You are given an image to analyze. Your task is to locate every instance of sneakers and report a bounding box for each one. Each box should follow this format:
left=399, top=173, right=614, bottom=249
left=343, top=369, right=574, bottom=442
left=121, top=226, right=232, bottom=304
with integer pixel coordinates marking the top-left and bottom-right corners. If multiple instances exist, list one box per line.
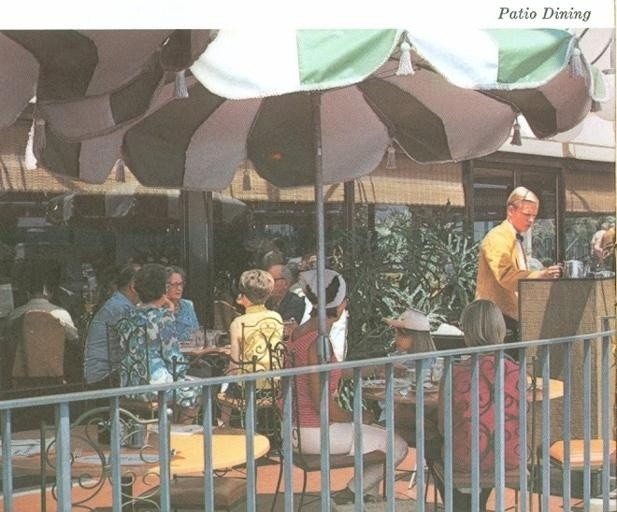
left=329, top=489, right=379, bottom=512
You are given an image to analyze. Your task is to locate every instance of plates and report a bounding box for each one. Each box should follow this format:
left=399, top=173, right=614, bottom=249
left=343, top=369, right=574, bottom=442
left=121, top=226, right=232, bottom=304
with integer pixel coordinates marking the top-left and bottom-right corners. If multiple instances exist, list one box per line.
left=359, top=370, right=439, bottom=396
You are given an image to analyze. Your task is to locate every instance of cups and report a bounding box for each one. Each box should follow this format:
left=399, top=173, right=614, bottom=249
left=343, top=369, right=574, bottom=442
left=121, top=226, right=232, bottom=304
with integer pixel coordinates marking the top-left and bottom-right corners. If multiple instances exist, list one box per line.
left=96, top=422, right=111, bottom=445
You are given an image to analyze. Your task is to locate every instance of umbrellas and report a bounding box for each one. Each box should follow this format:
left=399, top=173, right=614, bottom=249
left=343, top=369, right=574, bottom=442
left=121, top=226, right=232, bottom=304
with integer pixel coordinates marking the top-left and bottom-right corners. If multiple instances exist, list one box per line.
left=0, top=29, right=216, bottom=153
left=31, top=29, right=607, bottom=365
left=46, top=184, right=248, bottom=228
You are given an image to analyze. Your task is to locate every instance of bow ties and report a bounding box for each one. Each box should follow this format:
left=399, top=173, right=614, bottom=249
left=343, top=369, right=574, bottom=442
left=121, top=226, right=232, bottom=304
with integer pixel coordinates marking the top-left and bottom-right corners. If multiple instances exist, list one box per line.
left=515, top=232, right=525, bottom=242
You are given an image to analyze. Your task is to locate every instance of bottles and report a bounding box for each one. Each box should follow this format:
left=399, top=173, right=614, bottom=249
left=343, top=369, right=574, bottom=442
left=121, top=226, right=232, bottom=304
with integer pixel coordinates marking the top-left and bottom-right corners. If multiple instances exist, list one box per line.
left=431, top=358, right=445, bottom=384
left=128, top=414, right=147, bottom=449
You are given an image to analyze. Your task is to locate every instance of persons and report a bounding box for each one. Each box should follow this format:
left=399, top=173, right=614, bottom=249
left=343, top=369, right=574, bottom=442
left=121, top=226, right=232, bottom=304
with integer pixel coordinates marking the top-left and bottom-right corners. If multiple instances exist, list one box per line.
left=473, top=186, right=561, bottom=362
left=380, top=307, right=439, bottom=447
left=424, top=299, right=530, bottom=511
left=589, top=221, right=615, bottom=272
left=1, top=259, right=306, bottom=425
left=286, top=268, right=407, bottom=504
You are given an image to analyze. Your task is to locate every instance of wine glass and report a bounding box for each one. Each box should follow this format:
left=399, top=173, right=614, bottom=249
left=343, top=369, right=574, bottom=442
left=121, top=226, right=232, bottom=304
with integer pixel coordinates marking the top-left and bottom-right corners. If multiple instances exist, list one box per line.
left=183, top=325, right=217, bottom=352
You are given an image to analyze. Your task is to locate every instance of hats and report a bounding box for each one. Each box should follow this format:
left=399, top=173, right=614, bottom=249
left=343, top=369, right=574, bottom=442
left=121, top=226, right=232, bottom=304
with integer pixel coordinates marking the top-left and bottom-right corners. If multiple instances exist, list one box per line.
left=298, top=265, right=349, bottom=312
left=379, top=308, right=433, bottom=334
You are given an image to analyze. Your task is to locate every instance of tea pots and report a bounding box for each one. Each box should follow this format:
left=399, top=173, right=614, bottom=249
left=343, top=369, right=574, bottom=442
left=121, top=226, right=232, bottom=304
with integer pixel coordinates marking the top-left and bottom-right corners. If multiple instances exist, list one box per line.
left=553, top=260, right=590, bottom=278
